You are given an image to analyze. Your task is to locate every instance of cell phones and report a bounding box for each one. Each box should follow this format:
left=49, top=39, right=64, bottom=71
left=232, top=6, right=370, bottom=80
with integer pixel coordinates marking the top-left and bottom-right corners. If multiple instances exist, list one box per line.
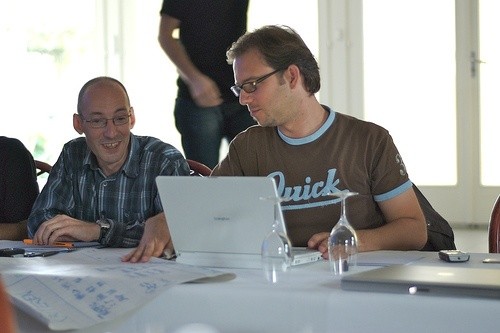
left=439, top=250, right=470, bottom=262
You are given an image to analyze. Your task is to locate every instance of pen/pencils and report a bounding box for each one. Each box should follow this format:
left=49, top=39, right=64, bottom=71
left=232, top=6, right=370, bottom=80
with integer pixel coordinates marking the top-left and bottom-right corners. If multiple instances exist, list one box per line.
left=22, top=239, right=74, bottom=247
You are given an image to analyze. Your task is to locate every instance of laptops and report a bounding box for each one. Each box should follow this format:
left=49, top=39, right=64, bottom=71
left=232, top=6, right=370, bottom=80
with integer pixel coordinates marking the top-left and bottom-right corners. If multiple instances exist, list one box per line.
left=340, top=264, right=500, bottom=299
left=156, top=175, right=325, bottom=267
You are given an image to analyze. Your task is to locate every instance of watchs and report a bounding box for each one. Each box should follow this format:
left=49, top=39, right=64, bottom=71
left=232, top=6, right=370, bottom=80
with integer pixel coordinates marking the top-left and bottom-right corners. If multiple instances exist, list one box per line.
left=96, top=218, right=110, bottom=243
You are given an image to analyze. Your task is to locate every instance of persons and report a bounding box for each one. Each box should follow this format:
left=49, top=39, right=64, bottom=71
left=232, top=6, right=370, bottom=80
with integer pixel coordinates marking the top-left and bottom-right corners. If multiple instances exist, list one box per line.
left=120, top=25, right=428, bottom=263
left=0, top=135, right=39, bottom=240
left=26, top=76, right=189, bottom=246
left=158, top=0, right=261, bottom=175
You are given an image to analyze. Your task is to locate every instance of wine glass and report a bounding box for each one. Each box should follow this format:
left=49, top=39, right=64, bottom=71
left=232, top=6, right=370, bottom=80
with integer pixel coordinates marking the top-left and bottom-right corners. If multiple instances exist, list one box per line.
left=257, top=195, right=294, bottom=270
left=322, top=191, right=360, bottom=277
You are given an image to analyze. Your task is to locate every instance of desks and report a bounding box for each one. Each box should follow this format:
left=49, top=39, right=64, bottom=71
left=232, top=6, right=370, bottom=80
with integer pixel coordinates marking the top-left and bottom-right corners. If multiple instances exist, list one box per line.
left=0, top=240, right=500, bottom=333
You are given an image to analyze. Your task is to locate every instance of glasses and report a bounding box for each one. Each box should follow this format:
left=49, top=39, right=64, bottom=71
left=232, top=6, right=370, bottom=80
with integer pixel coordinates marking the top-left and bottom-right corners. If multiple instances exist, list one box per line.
left=230, top=67, right=290, bottom=97
left=81, top=110, right=132, bottom=128
